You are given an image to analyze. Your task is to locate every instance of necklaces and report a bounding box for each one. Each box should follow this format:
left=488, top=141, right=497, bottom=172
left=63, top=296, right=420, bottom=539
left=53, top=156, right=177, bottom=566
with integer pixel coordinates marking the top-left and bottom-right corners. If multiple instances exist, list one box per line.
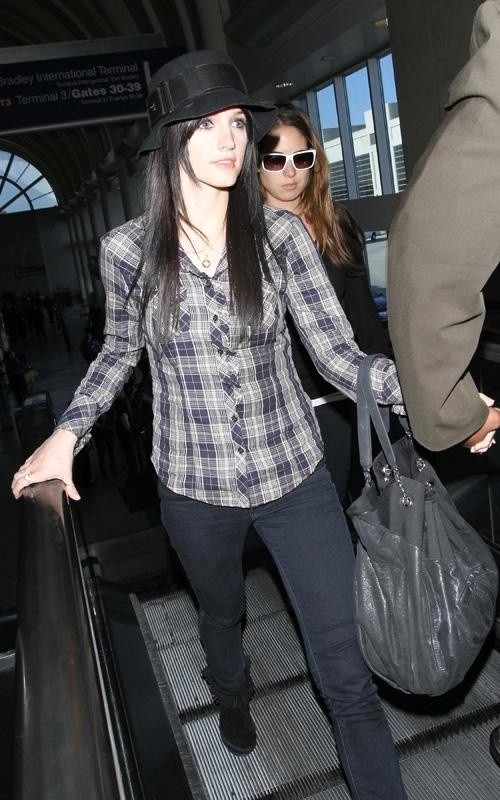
left=200, top=228, right=223, bottom=269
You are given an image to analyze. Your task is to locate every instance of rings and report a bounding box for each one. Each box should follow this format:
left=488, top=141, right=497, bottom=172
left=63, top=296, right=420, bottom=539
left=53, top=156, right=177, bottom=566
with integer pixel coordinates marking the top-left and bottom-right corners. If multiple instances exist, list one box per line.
left=24, top=472, right=33, bottom=482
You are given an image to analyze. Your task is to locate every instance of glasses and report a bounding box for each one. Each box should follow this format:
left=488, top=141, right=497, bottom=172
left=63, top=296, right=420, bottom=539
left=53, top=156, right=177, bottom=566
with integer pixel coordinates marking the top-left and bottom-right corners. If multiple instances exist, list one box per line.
left=259, top=147, right=319, bottom=174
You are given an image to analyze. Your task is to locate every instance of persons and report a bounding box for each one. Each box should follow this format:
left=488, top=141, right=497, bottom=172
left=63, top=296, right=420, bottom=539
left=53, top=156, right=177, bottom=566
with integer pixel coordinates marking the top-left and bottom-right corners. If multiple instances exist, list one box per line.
left=382, top=1, right=499, bottom=767
left=80, top=362, right=153, bottom=488
left=257, top=100, right=390, bottom=510
left=80, top=331, right=103, bottom=365
left=11, top=47, right=497, bottom=799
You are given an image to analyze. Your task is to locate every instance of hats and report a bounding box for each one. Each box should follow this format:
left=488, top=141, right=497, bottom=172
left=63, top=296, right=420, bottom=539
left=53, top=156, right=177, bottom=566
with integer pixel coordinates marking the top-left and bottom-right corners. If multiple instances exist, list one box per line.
left=133, top=46, right=278, bottom=161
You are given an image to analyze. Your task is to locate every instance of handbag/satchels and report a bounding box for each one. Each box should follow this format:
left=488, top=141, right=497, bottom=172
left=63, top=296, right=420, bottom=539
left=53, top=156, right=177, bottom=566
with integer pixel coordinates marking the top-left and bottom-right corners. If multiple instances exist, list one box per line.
left=346, top=351, right=498, bottom=700
